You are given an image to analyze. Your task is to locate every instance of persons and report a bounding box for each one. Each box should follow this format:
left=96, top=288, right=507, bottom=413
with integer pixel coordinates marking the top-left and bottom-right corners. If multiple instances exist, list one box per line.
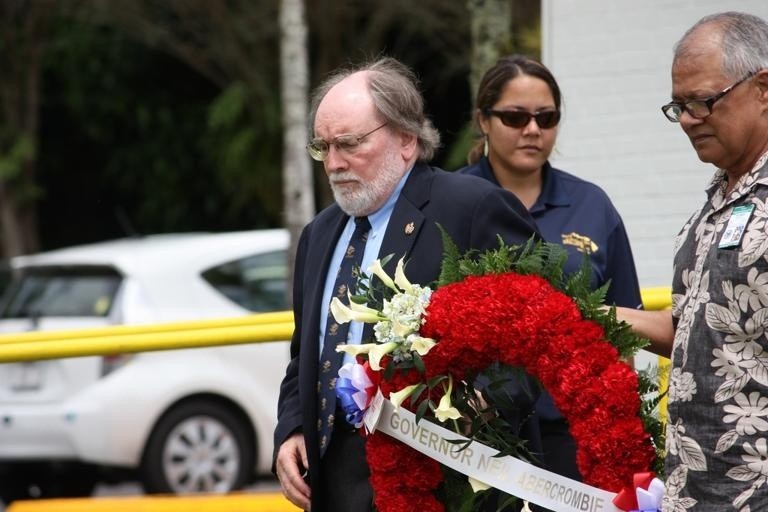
left=448, top=55, right=645, bottom=512
left=575, top=14, right=768, bottom=512
left=273, top=60, right=584, bottom=512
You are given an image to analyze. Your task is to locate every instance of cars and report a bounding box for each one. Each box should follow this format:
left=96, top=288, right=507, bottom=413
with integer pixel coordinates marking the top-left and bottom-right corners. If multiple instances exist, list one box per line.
left=0, top=229, right=290, bottom=498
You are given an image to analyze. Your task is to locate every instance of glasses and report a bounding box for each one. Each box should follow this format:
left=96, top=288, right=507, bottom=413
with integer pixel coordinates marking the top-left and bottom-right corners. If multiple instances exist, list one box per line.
left=661, top=72, right=757, bottom=123
left=305, top=121, right=390, bottom=161
left=488, top=109, right=562, bottom=129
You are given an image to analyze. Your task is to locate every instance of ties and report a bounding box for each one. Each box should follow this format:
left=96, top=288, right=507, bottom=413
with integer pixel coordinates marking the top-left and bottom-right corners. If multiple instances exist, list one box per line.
left=318, top=215, right=372, bottom=458
